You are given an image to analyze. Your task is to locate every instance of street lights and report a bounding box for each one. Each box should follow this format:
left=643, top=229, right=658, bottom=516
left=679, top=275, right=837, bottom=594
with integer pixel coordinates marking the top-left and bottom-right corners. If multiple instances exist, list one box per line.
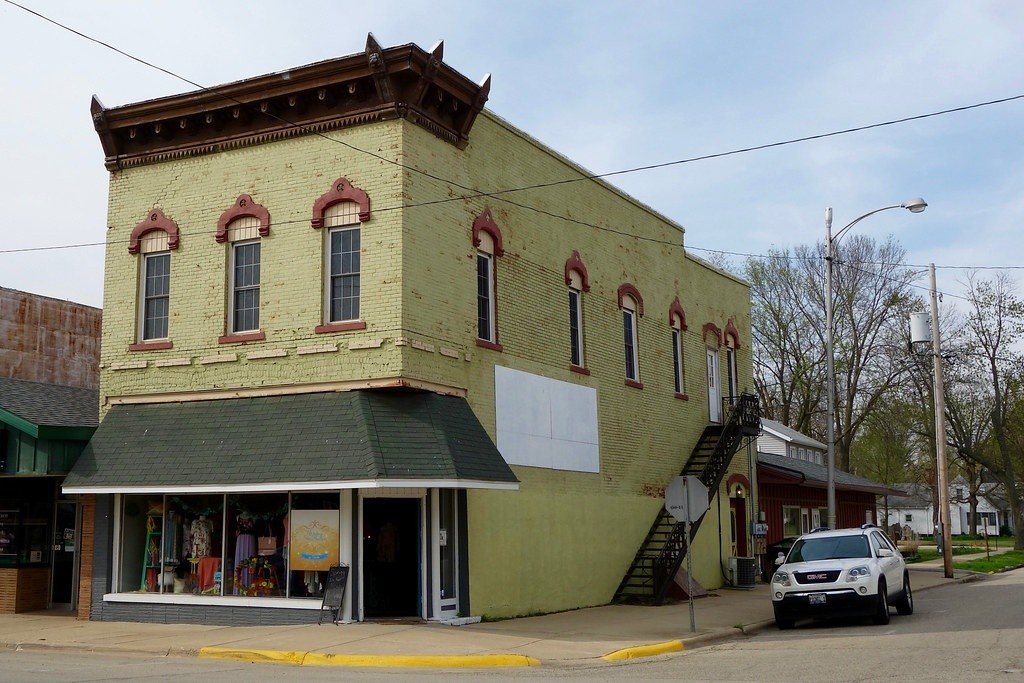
left=826, top=198, right=928, bottom=532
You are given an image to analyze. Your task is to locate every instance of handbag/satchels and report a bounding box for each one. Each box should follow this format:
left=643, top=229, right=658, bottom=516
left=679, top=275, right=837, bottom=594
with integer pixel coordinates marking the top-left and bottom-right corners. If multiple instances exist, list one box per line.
left=258, top=522, right=277, bottom=556
left=158, top=572, right=177, bottom=585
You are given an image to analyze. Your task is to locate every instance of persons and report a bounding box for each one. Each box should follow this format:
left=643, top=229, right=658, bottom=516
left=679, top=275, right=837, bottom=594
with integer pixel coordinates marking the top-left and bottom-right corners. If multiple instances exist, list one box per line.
left=233, top=510, right=256, bottom=595
left=182, top=518, right=192, bottom=558
left=191, top=515, right=213, bottom=558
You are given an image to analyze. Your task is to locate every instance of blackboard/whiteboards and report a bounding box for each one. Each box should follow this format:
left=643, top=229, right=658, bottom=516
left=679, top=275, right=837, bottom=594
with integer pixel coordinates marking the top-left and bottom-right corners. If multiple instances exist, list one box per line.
left=322, top=567, right=350, bottom=606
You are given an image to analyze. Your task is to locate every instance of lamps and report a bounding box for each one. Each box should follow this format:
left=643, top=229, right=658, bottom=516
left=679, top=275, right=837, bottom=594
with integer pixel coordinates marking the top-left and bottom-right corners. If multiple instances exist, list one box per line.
left=737, top=488, right=742, bottom=494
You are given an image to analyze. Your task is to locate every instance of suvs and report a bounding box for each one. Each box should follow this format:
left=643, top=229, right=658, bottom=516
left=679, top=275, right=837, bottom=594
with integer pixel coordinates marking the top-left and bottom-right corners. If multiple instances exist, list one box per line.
left=770, top=522, right=913, bottom=631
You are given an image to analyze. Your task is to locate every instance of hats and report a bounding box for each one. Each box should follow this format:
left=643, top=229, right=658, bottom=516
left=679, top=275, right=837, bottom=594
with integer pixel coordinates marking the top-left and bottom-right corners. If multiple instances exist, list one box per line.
left=240, top=512, right=252, bottom=519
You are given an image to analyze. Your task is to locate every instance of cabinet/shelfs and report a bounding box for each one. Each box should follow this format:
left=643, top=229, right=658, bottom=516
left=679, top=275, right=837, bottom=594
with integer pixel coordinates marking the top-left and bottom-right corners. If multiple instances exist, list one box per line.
left=0, top=508, right=24, bottom=563
